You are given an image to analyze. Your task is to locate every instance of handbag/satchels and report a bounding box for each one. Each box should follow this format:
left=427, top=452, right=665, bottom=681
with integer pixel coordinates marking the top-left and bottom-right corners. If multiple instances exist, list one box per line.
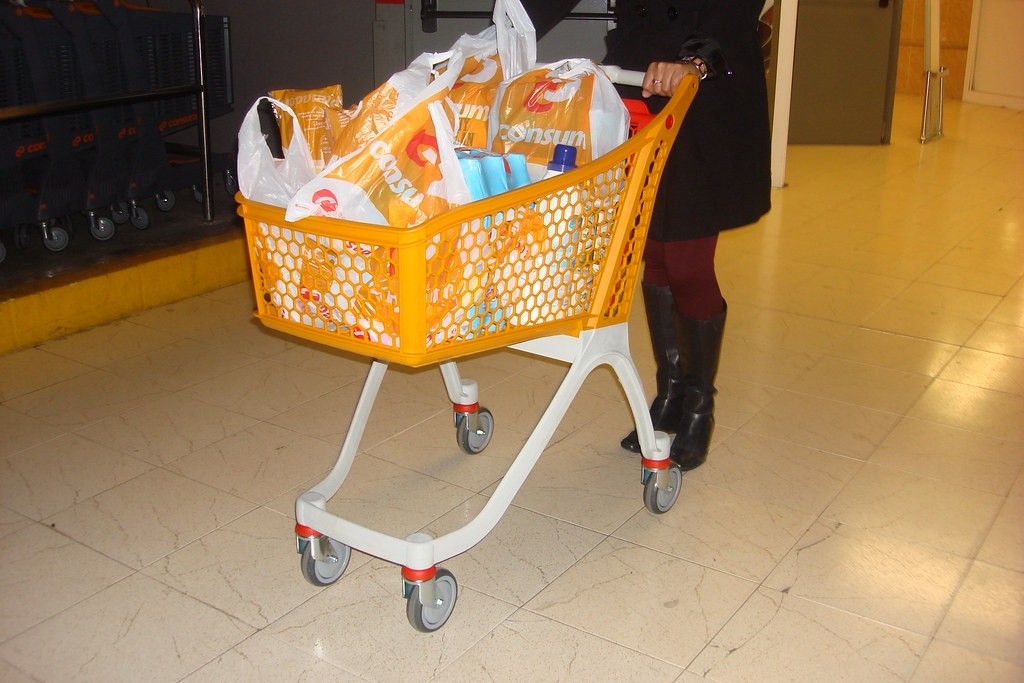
left=238, top=0, right=631, bottom=350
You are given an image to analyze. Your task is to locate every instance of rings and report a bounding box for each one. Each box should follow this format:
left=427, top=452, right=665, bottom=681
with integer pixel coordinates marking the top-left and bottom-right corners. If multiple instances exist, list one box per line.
left=652, top=79, right=662, bottom=85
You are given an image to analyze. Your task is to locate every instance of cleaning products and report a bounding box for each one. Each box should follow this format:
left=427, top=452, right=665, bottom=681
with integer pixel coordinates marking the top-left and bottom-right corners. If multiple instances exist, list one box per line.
left=526, top=143, right=589, bottom=327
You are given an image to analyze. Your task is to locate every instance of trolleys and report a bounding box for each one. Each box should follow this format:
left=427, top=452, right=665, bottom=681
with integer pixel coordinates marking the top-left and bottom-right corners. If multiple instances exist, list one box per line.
left=234, top=62, right=700, bottom=635
left=1, top=0, right=242, bottom=262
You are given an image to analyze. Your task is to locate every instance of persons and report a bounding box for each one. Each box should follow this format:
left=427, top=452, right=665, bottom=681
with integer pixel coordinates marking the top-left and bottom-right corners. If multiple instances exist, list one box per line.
left=520, top=0, right=773, bottom=469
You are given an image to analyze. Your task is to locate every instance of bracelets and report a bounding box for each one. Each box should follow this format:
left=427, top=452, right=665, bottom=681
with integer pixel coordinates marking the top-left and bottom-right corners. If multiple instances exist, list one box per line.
left=681, top=55, right=708, bottom=80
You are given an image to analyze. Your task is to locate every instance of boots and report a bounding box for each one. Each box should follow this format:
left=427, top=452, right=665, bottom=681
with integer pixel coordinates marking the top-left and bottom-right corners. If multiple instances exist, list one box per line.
left=670, top=298, right=728, bottom=472
left=620, top=282, right=682, bottom=452
